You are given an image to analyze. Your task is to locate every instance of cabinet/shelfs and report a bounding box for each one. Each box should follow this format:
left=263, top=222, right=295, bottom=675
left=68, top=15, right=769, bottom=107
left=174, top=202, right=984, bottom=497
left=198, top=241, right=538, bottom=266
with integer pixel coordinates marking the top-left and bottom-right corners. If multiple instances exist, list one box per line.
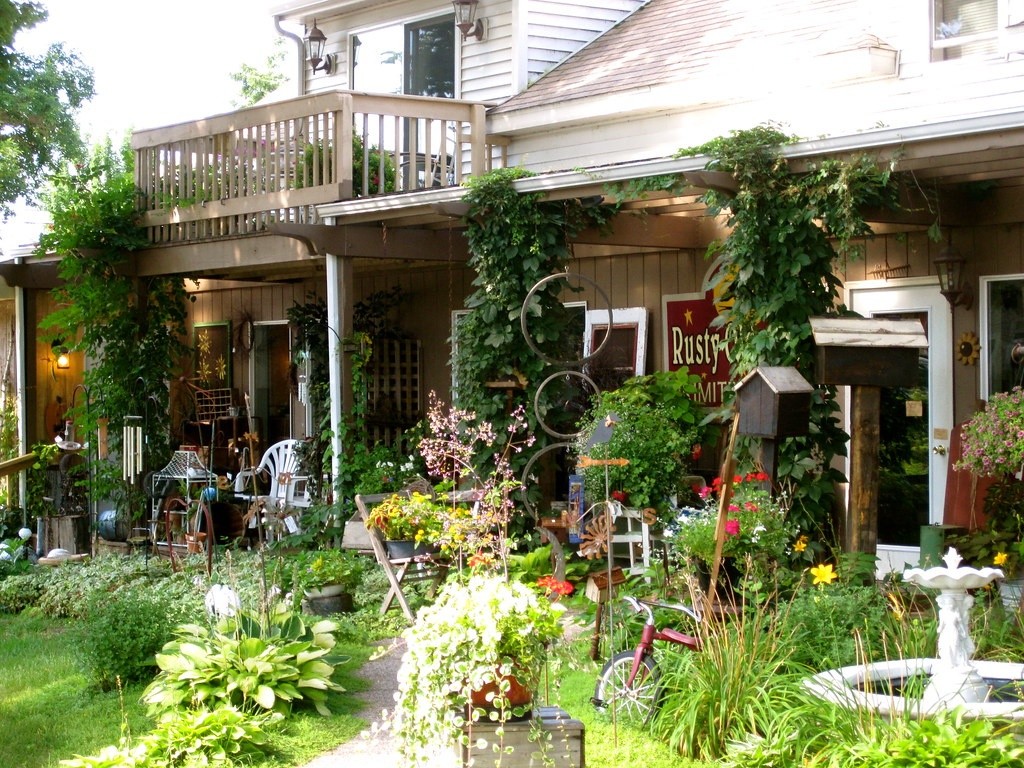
left=605, top=504, right=664, bottom=588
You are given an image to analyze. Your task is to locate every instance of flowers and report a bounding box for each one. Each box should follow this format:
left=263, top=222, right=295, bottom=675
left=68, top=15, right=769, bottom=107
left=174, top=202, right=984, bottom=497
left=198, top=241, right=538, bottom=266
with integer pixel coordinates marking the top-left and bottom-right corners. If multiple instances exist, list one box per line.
left=946, top=384, right=1024, bottom=478
left=366, top=490, right=467, bottom=554
left=678, top=470, right=792, bottom=558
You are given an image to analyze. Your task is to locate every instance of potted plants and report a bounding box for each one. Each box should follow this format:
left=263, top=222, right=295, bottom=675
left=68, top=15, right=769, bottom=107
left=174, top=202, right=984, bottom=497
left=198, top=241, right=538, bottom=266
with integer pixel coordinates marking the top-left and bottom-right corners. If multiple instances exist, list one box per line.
left=75, top=456, right=137, bottom=542
left=540, top=508, right=568, bottom=543
left=229, top=400, right=240, bottom=416
left=980, top=475, right=1024, bottom=567
left=361, top=570, right=572, bottom=768
left=297, top=553, right=367, bottom=615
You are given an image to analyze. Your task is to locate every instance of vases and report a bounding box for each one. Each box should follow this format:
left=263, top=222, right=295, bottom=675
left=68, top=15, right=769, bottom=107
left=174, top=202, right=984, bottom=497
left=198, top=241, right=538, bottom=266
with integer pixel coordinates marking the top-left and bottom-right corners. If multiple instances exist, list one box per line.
left=187, top=532, right=207, bottom=553
left=383, top=539, right=440, bottom=559
left=696, top=554, right=746, bottom=603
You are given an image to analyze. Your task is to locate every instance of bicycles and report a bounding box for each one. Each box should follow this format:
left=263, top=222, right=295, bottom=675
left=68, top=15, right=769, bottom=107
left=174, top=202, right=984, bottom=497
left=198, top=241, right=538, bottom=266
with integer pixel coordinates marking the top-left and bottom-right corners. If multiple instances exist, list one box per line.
left=589, top=595, right=786, bottom=744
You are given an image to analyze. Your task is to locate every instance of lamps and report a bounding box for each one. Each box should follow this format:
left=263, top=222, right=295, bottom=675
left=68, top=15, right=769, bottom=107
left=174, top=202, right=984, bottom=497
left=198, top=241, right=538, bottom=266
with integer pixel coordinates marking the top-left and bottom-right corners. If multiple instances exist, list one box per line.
left=57, top=383, right=111, bottom=563
left=933, top=242, right=974, bottom=313
left=49, top=333, right=70, bottom=382
left=451, top=0, right=489, bottom=44
left=304, top=17, right=335, bottom=77
left=806, top=33, right=902, bottom=87
left=117, top=375, right=149, bottom=557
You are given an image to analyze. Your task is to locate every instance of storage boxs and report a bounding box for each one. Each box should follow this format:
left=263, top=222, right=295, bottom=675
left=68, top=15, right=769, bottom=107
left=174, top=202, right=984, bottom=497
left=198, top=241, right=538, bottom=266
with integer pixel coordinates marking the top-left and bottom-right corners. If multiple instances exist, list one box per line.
left=450, top=704, right=585, bottom=768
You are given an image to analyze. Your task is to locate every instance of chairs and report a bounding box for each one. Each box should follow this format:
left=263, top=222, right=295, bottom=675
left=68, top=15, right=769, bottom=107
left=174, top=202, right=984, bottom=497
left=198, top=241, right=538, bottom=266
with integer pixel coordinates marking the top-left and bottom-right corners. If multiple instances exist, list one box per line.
left=285, top=453, right=414, bottom=539
left=388, top=116, right=458, bottom=191
left=233, top=438, right=303, bottom=544
